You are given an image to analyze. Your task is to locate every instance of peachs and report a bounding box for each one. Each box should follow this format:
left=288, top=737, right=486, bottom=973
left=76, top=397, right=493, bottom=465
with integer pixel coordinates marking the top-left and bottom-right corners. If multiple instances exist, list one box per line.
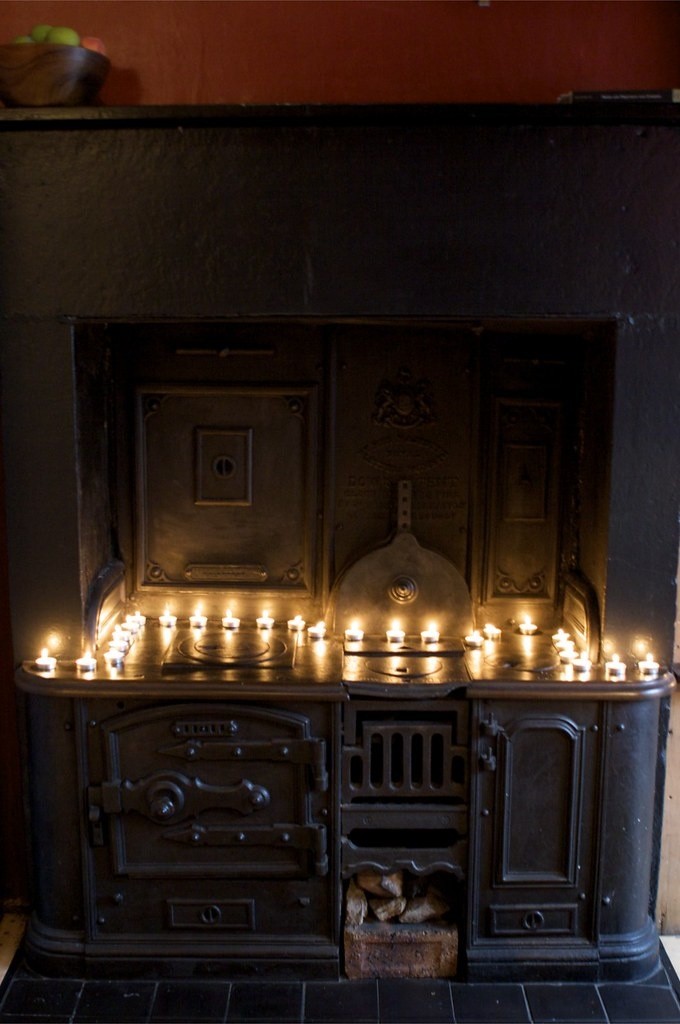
left=78, top=37, right=107, bottom=56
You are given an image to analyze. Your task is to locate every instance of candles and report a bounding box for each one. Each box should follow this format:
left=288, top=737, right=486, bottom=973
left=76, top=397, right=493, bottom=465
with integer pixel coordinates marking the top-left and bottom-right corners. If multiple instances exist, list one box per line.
left=638, top=653, right=659, bottom=674
left=345, top=621, right=363, bottom=641
left=104, top=612, right=146, bottom=665
left=420, top=623, right=439, bottom=643
left=189, top=610, right=207, bottom=627
left=35, top=648, right=56, bottom=670
left=605, top=653, right=626, bottom=677
left=386, top=620, right=405, bottom=643
left=76, top=650, right=96, bottom=670
left=519, top=617, right=536, bottom=635
left=288, top=615, right=305, bottom=630
left=222, top=610, right=240, bottom=628
left=159, top=611, right=177, bottom=626
left=484, top=623, right=501, bottom=639
left=255, top=611, right=274, bottom=629
left=308, top=621, right=326, bottom=637
left=552, top=628, right=591, bottom=672
left=465, top=630, right=484, bottom=646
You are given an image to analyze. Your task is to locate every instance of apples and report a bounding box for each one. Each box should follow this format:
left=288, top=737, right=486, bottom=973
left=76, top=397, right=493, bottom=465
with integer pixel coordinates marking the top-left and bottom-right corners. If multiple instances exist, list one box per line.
left=12, top=22, right=81, bottom=46
